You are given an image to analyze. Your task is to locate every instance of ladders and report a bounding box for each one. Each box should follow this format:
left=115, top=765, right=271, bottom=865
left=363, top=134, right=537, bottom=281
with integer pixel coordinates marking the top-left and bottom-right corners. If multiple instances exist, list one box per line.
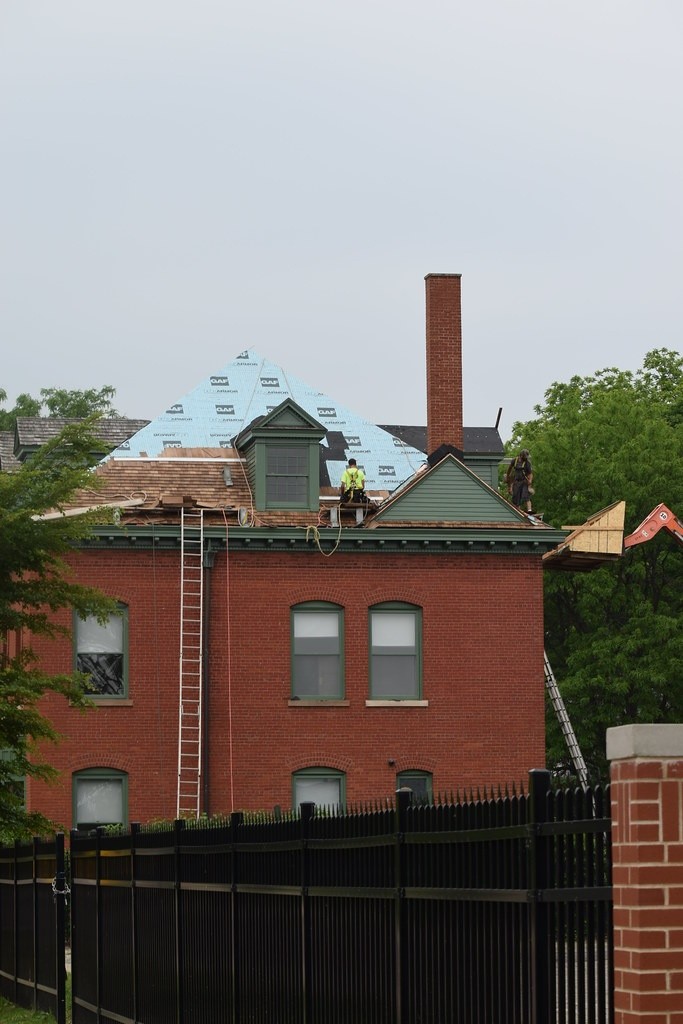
left=544, top=649, right=597, bottom=818
left=177, top=506, right=203, bottom=820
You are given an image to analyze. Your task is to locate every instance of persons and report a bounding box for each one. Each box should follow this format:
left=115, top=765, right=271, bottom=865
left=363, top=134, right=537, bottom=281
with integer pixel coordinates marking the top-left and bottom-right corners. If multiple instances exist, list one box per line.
left=340, top=459, right=365, bottom=501
left=507, top=449, right=533, bottom=514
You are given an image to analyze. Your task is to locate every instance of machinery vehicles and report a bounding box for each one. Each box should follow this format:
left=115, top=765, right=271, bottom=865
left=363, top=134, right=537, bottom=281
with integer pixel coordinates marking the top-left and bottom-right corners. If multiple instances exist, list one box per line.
left=543, top=503, right=683, bottom=569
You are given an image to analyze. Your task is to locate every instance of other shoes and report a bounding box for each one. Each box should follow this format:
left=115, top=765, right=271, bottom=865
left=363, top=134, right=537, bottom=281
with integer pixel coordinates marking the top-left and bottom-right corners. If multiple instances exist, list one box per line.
left=528, top=510, right=537, bottom=515
